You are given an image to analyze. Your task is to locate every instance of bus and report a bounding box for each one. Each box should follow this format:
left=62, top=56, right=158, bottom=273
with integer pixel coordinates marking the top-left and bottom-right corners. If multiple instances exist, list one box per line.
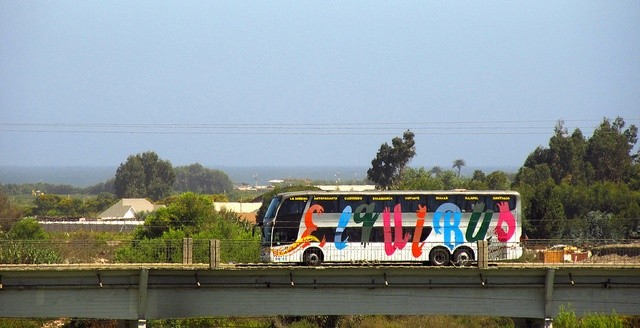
left=251, top=189, right=524, bottom=266
